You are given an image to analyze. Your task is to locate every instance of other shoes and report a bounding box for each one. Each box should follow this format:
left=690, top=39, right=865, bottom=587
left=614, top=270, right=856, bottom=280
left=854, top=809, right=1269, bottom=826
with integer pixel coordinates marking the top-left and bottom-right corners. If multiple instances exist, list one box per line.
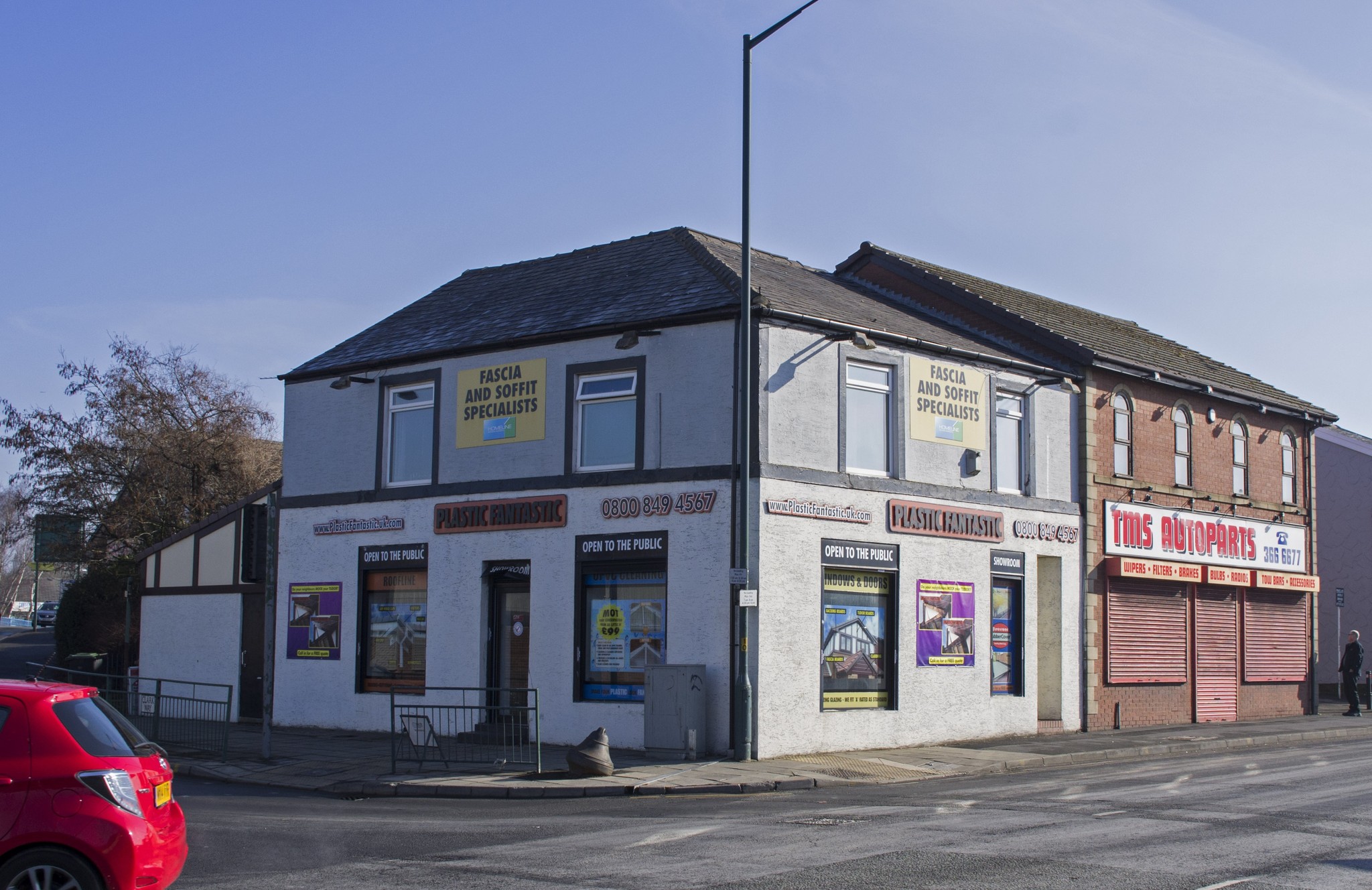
left=1342, top=709, right=1362, bottom=717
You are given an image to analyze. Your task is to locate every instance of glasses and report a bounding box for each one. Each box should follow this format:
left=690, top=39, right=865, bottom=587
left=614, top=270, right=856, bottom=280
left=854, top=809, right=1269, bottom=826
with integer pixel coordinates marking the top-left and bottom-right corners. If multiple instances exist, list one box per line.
left=1348, top=633, right=1354, bottom=636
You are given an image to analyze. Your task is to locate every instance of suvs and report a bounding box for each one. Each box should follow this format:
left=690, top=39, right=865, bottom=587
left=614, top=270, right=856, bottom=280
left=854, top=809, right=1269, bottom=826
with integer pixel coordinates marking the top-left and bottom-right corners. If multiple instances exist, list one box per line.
left=31, top=600, right=60, bottom=627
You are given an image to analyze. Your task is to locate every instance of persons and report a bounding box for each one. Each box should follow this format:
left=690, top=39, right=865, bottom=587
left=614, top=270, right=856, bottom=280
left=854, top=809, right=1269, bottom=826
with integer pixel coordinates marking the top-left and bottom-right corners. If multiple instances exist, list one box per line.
left=1337, top=630, right=1364, bottom=716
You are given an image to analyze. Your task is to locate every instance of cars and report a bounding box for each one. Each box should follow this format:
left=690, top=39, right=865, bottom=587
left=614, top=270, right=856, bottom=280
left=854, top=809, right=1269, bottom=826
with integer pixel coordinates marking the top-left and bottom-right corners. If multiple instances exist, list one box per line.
left=0, top=676, right=186, bottom=890
